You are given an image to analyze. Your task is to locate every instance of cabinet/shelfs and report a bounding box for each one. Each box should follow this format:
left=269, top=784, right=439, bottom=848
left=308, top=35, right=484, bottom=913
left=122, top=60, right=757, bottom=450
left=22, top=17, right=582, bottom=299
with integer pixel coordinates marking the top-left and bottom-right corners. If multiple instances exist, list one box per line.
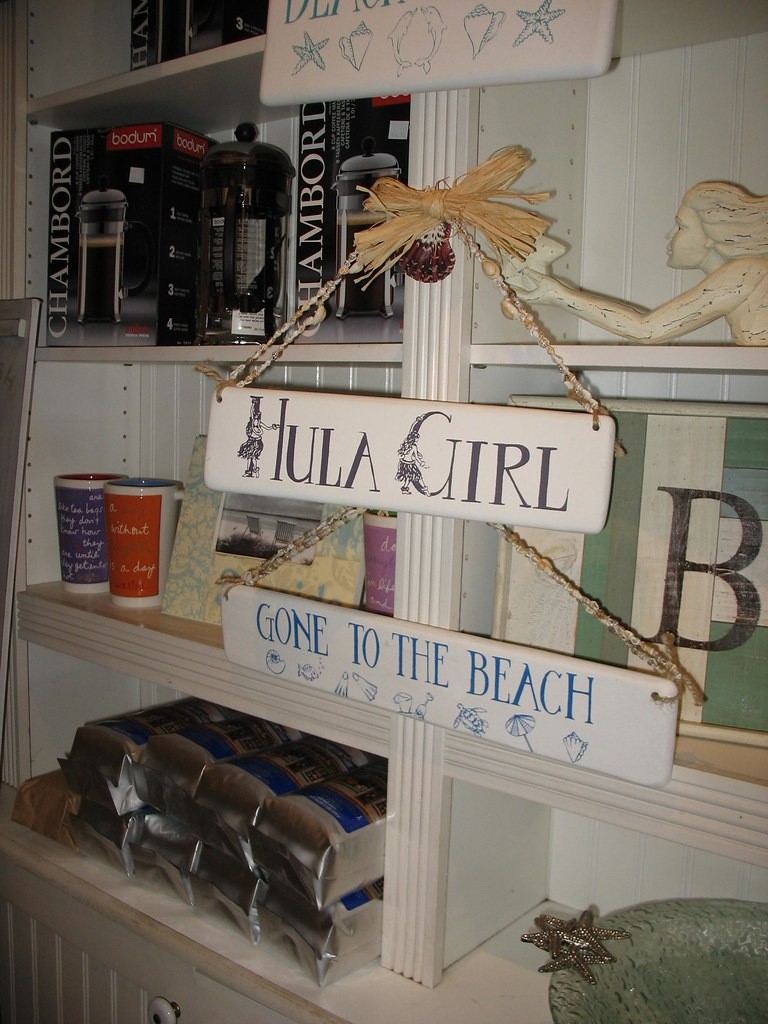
left=0, top=0, right=768, bottom=1024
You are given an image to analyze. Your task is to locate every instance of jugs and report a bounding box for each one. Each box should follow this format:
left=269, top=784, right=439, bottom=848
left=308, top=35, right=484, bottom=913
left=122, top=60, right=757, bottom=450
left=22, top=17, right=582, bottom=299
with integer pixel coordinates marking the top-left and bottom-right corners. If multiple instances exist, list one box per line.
left=193, top=122, right=296, bottom=344
left=332, top=150, right=402, bottom=319
left=75, top=187, right=127, bottom=324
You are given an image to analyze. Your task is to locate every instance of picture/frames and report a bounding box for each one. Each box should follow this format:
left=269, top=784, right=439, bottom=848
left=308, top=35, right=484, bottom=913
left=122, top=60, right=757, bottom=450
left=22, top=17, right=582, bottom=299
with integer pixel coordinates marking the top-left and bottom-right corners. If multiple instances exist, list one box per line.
left=162, top=436, right=367, bottom=626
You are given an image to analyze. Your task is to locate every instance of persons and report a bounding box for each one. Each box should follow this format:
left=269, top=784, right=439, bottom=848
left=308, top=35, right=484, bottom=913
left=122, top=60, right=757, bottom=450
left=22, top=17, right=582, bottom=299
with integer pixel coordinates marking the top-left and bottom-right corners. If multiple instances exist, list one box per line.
left=511, top=179, right=768, bottom=344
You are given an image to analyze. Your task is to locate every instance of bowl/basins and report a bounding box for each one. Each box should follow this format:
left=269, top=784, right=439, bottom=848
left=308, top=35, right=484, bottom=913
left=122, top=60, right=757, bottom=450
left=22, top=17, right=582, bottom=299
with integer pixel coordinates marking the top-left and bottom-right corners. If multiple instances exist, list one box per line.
left=549, top=896, right=768, bottom=1024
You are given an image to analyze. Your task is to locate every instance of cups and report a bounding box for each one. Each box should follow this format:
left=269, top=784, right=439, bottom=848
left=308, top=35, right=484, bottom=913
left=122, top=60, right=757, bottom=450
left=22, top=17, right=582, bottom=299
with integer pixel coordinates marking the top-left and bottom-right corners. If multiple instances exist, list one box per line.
left=363, top=510, right=398, bottom=617
left=103, top=478, right=186, bottom=610
left=54, top=472, right=130, bottom=592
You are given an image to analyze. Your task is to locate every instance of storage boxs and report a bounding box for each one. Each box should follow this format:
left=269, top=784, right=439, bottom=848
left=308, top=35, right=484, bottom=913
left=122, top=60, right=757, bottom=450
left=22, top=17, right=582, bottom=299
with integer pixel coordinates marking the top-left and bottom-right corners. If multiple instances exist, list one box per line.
left=186, top=0, right=269, bottom=54
left=295, top=93, right=410, bottom=344
left=45, top=121, right=220, bottom=345
left=131, top=0, right=187, bottom=72
